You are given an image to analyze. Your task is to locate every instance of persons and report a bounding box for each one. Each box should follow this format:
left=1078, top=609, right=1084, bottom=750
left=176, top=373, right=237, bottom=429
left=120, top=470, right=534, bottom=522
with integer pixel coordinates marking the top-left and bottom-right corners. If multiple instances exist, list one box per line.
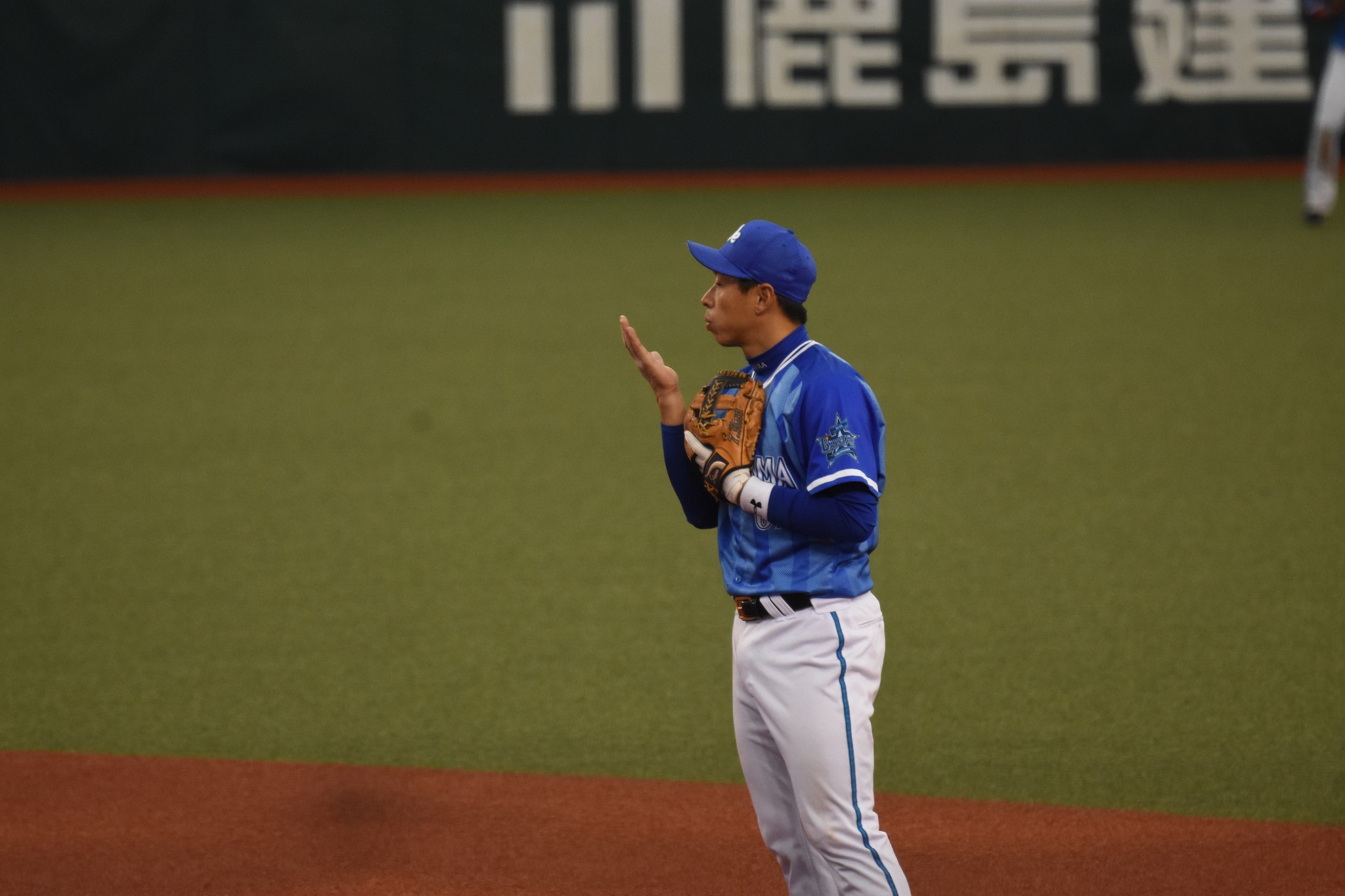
left=1304, top=1, right=1345, bottom=224
left=620, top=220, right=915, bottom=896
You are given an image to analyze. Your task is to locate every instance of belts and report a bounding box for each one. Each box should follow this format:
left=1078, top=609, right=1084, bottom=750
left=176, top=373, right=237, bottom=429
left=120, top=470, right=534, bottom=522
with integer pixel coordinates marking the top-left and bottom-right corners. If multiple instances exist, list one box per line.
left=735, top=594, right=813, bottom=621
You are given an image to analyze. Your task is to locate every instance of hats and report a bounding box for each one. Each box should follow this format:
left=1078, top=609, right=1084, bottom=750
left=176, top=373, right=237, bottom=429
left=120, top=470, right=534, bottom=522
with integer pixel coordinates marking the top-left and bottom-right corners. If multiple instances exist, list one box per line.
left=687, top=220, right=817, bottom=303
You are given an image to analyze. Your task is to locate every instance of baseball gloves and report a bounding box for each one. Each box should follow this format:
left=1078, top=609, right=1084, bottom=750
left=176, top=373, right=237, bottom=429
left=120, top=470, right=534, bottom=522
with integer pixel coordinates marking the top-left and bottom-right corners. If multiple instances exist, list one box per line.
left=683, top=368, right=767, bottom=503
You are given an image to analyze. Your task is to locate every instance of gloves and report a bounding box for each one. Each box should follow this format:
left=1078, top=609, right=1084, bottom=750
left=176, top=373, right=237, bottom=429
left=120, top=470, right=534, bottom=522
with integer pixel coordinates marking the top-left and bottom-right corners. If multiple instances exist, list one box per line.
left=684, top=430, right=750, bottom=504
